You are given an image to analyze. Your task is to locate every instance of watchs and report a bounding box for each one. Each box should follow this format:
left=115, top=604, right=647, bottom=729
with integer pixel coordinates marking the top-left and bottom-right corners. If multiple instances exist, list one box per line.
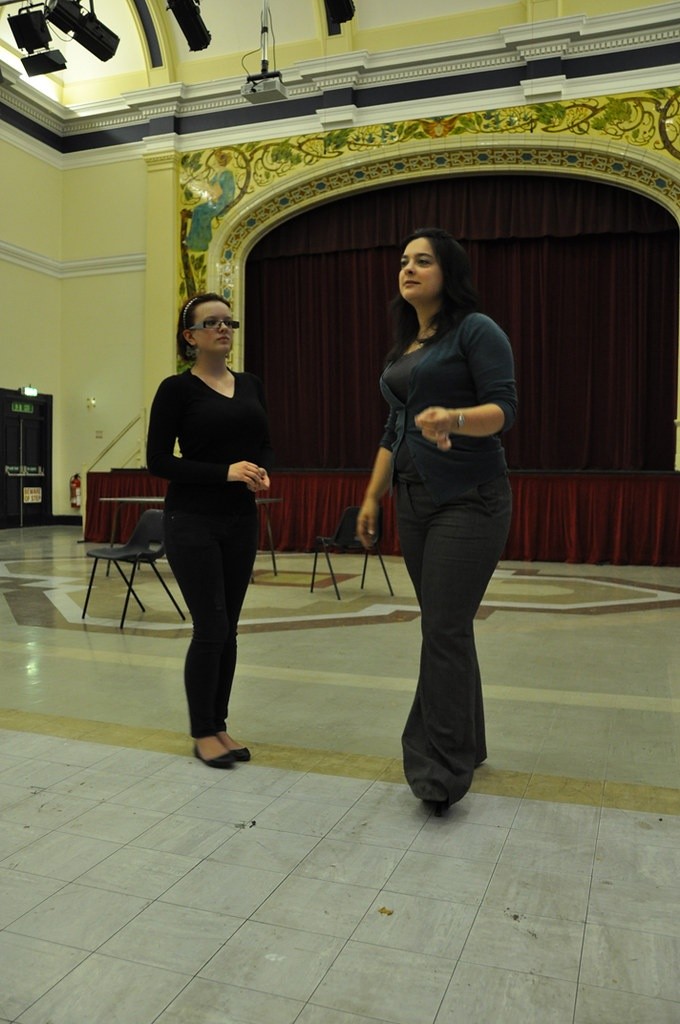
left=452, top=408, right=465, bottom=431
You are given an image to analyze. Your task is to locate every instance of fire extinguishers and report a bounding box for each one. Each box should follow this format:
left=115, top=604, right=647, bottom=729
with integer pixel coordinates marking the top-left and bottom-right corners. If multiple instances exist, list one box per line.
left=70, top=473, right=81, bottom=509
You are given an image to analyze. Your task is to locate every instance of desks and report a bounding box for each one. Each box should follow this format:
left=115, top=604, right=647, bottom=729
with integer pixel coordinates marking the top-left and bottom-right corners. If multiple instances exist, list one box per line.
left=97, top=497, right=285, bottom=585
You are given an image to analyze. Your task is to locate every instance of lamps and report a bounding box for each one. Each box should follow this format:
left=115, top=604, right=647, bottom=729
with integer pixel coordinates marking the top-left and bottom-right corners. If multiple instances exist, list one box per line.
left=43, top=0, right=120, bottom=62
left=324, top=0, right=355, bottom=24
left=8, top=3, right=66, bottom=77
left=167, top=0, right=211, bottom=51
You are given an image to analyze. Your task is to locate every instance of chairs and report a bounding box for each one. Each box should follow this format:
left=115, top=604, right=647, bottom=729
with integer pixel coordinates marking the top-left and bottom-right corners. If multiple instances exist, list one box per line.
left=82, top=510, right=187, bottom=629
left=310, top=505, right=395, bottom=600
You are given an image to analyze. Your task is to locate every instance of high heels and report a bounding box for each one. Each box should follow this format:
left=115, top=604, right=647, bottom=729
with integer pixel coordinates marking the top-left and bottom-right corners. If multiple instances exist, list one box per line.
left=420, top=798, right=450, bottom=817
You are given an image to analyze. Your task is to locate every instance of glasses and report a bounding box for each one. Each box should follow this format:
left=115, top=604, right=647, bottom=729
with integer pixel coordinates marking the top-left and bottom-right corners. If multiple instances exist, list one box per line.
left=189, top=319, right=240, bottom=331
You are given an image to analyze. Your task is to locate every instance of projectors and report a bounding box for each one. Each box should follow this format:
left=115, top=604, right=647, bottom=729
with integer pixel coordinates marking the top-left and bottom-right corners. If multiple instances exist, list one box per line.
left=241, top=80, right=288, bottom=103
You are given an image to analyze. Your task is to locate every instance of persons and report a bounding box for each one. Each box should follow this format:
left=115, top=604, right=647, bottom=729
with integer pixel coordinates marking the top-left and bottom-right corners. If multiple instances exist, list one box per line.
left=147, top=295, right=271, bottom=768
left=359, top=229, right=517, bottom=814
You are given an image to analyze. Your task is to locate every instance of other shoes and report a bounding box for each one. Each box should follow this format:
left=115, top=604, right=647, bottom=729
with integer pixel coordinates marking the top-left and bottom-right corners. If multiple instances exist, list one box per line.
left=193, top=742, right=237, bottom=768
left=230, top=746, right=251, bottom=763
